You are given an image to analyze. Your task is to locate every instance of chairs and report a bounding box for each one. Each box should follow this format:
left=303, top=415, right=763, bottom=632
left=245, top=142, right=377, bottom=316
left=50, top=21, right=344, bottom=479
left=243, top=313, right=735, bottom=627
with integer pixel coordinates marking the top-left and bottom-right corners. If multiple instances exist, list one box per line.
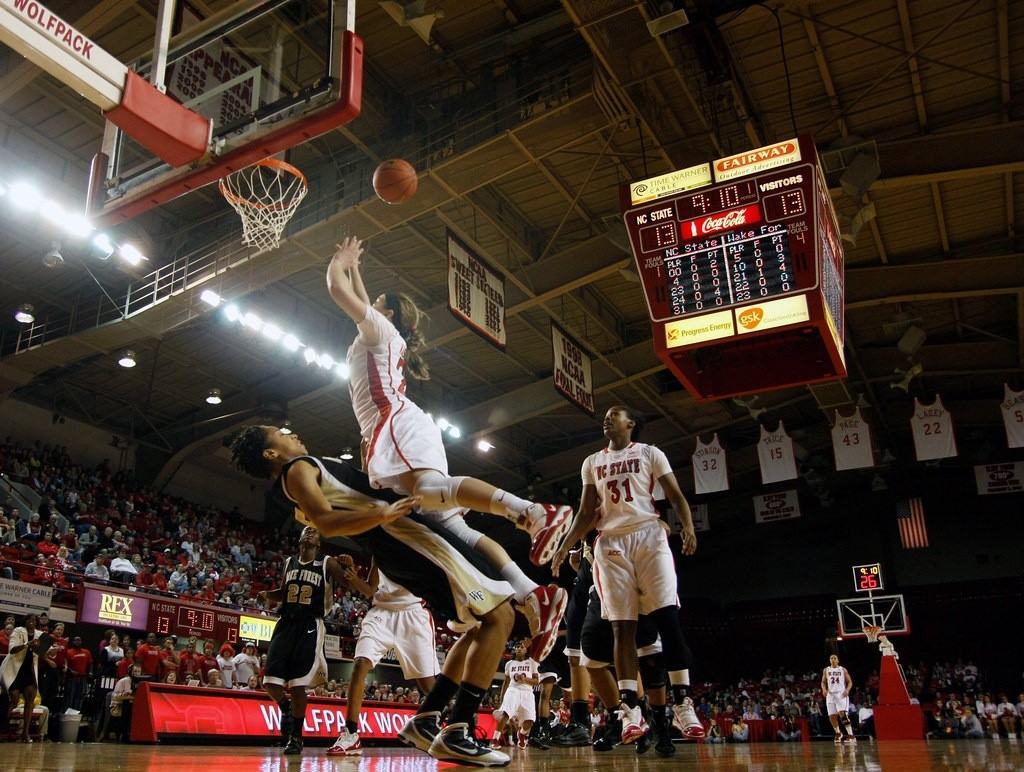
left=97, top=691, right=124, bottom=745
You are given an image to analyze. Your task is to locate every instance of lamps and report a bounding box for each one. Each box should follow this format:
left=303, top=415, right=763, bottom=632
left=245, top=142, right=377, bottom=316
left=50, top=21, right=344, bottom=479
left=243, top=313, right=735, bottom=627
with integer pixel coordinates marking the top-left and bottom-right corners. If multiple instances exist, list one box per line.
left=13, top=230, right=142, bottom=369
left=278, top=420, right=294, bottom=434
left=205, top=388, right=222, bottom=404
left=340, top=447, right=354, bottom=460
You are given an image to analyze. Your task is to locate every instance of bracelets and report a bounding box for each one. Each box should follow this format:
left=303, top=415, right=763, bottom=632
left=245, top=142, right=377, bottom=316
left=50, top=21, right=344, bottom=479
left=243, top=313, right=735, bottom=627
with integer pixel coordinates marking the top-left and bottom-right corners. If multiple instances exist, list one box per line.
left=24, top=642, right=29, bottom=648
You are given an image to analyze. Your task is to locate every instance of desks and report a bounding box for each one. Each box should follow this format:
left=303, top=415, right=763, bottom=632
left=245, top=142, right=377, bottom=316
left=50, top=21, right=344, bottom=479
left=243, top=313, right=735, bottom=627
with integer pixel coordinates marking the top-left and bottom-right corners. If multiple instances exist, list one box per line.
left=698, top=718, right=811, bottom=743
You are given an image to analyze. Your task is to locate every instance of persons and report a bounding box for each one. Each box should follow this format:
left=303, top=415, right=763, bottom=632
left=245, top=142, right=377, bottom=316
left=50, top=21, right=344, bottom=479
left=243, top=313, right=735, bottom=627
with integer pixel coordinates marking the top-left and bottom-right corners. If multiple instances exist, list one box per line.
left=489, top=639, right=539, bottom=750
left=528, top=405, right=706, bottom=760
left=257, top=526, right=358, bottom=756
left=326, top=234, right=573, bottom=665
left=665, top=657, right=1024, bottom=739
left=0, top=438, right=609, bottom=744
left=821, top=654, right=857, bottom=743
left=229, top=424, right=517, bottom=770
left=325, top=552, right=478, bottom=757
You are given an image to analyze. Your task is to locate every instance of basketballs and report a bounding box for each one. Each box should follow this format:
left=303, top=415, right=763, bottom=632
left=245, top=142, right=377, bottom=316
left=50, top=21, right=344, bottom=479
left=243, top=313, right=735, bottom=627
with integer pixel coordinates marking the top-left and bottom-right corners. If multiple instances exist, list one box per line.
left=372, top=158, right=418, bottom=205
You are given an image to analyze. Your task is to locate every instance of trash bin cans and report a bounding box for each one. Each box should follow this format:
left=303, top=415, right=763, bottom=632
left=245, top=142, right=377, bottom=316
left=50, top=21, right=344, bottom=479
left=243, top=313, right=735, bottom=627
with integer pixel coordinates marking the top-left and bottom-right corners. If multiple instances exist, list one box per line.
left=58, top=708, right=83, bottom=743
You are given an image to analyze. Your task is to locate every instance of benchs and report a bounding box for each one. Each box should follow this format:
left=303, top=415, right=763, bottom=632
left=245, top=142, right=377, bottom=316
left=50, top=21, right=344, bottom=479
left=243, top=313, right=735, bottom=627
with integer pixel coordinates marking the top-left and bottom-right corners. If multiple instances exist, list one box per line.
left=0, top=712, right=44, bottom=742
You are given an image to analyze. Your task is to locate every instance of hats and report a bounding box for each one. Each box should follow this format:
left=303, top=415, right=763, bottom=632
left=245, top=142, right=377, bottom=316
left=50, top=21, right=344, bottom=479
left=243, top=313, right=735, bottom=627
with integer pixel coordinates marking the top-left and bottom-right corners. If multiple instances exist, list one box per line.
left=219, top=644, right=235, bottom=657
left=48, top=555, right=56, bottom=560
left=164, top=549, right=170, bottom=552
left=246, top=642, right=255, bottom=647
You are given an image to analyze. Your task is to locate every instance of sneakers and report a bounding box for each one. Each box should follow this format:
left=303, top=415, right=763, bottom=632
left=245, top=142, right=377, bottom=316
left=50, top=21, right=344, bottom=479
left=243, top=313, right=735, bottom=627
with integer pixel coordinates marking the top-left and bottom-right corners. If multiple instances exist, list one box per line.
left=427, top=723, right=510, bottom=768
left=550, top=722, right=592, bottom=747
left=524, top=731, right=530, bottom=744
left=593, top=719, right=624, bottom=751
left=672, top=697, right=705, bottom=740
left=834, top=732, right=843, bottom=743
left=647, top=707, right=676, bottom=756
left=514, top=584, right=568, bottom=662
left=281, top=698, right=294, bottom=734
left=517, top=730, right=526, bottom=750
left=538, top=717, right=551, bottom=744
left=516, top=502, right=573, bottom=565
left=844, top=735, right=858, bottom=743
left=325, top=726, right=363, bottom=756
left=489, top=738, right=501, bottom=750
left=283, top=735, right=304, bottom=755
left=614, top=703, right=649, bottom=745
left=397, top=712, right=441, bottom=752
